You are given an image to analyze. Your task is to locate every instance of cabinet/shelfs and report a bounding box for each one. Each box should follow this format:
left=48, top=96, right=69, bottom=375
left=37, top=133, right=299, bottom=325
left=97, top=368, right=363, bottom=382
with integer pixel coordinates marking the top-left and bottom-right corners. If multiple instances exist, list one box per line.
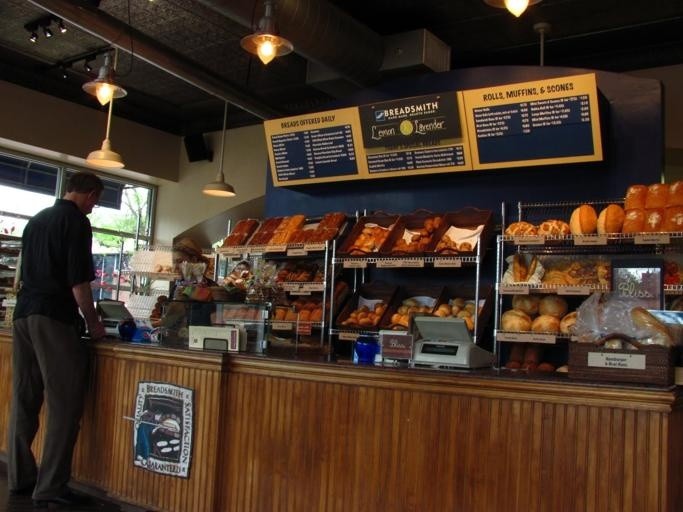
left=332, top=200, right=507, bottom=371
left=493, top=194, right=683, bottom=372
left=213, top=208, right=360, bottom=356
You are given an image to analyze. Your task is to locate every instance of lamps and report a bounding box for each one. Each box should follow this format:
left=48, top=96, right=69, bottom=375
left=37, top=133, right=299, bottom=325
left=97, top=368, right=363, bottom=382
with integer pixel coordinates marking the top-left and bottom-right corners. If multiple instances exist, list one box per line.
left=485, top=1, right=542, bottom=18
left=240, top=0, right=295, bottom=65
left=82, top=47, right=127, bottom=106
left=24, top=14, right=68, bottom=43
left=201, top=101, right=237, bottom=198
left=61, top=53, right=96, bottom=79
left=86, top=44, right=126, bottom=169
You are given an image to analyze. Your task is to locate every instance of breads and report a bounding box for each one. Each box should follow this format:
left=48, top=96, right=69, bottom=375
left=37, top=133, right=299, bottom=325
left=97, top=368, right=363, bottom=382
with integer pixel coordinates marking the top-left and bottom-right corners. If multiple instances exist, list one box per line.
left=392, top=217, right=442, bottom=250
left=393, top=297, right=434, bottom=328
left=537, top=220, right=570, bottom=235
left=514, top=255, right=521, bottom=282
left=349, top=227, right=391, bottom=252
left=341, top=303, right=387, bottom=328
left=560, top=311, right=576, bottom=332
left=643, top=207, right=668, bottom=232
left=622, top=208, right=647, bottom=233
left=504, top=223, right=537, bottom=235
left=625, top=185, right=646, bottom=210
left=644, top=184, right=669, bottom=209
left=665, top=206, right=683, bottom=232
left=570, top=205, right=597, bottom=234
left=521, top=254, right=526, bottom=281
left=502, top=309, right=531, bottom=329
left=596, top=204, right=626, bottom=233
left=441, top=234, right=472, bottom=249
left=631, top=307, right=672, bottom=337
left=667, top=181, right=683, bottom=208
left=530, top=315, right=560, bottom=331
left=526, top=256, right=538, bottom=282
left=511, top=294, right=542, bottom=314
left=539, top=296, right=568, bottom=318
left=436, top=296, right=477, bottom=330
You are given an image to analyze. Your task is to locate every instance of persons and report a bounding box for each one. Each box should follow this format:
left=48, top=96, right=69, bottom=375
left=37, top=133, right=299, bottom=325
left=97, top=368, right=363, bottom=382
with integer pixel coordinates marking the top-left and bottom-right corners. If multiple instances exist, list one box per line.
left=8, top=172, right=106, bottom=512
left=150, top=237, right=212, bottom=346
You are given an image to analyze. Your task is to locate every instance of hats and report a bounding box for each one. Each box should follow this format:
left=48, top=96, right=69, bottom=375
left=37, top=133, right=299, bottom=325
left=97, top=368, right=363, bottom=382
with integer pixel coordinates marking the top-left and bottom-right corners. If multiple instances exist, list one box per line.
left=172, top=238, right=204, bottom=260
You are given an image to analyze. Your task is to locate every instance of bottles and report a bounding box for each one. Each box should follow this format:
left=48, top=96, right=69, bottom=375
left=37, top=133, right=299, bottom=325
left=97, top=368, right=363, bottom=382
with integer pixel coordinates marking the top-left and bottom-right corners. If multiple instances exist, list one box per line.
left=352, top=330, right=381, bottom=364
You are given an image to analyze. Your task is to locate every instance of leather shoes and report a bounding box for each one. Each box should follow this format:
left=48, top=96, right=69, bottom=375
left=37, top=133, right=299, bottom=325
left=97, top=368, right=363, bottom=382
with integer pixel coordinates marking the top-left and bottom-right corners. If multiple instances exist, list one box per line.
left=8, top=484, right=35, bottom=502
left=30, top=486, right=92, bottom=508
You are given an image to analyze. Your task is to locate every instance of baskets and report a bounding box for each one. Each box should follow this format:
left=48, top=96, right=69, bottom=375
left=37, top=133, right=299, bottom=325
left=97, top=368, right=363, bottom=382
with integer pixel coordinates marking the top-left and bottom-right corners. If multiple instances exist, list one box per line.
left=375, top=277, right=449, bottom=331
left=430, top=279, right=496, bottom=338
left=566, top=332, right=676, bottom=389
left=334, top=277, right=402, bottom=331
left=335, top=208, right=401, bottom=258
left=422, top=206, right=494, bottom=258
left=378, top=207, right=446, bottom=258
